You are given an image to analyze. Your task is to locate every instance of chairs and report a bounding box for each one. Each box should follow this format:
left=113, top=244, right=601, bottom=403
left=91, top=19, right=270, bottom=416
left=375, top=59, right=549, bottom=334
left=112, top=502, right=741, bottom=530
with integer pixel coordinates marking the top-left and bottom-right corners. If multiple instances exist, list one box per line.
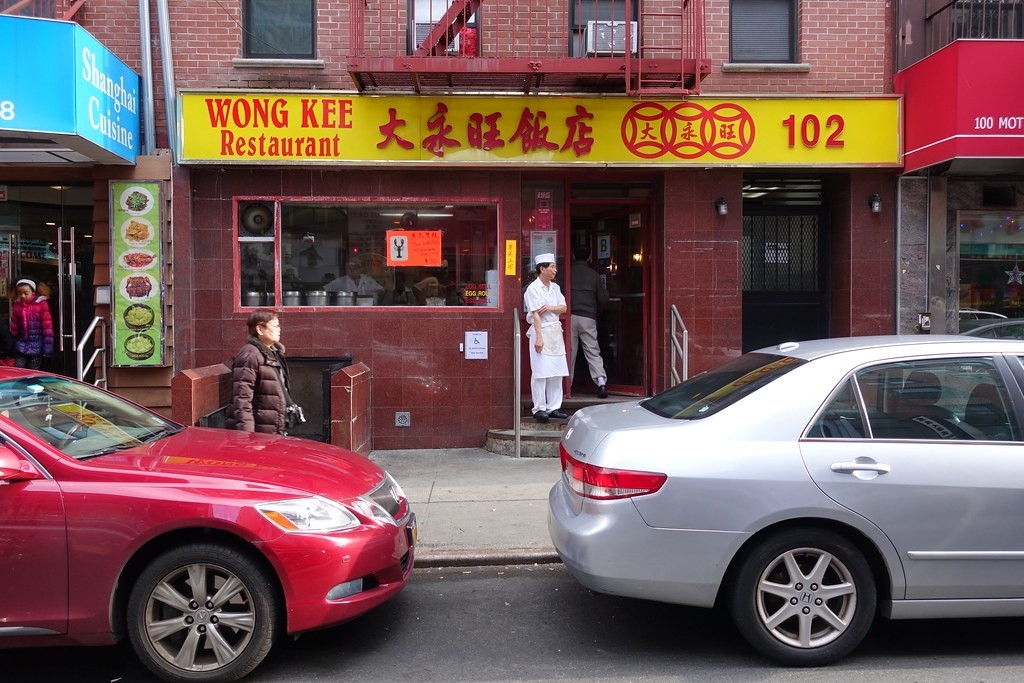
left=887, top=371, right=952, bottom=422
left=958, top=382, right=1016, bottom=440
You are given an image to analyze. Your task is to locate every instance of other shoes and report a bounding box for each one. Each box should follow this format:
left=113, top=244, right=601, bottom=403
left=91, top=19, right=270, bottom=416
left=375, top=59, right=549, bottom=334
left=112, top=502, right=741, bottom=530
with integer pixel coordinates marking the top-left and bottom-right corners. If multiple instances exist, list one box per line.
left=597, top=385, right=608, bottom=398
left=533, top=410, right=547, bottom=420
left=548, top=409, right=568, bottom=419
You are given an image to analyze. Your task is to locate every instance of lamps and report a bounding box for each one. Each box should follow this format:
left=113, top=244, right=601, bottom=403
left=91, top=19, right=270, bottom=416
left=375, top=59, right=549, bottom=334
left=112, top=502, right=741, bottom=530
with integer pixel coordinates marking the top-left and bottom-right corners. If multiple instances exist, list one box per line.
left=714, top=196, right=729, bottom=215
left=868, top=193, right=882, bottom=212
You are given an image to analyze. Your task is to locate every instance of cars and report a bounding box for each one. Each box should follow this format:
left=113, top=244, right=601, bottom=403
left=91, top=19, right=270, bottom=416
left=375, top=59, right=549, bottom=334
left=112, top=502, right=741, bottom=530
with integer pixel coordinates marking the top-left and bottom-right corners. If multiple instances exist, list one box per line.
left=547, top=334, right=1024, bottom=670
left=958, top=309, right=1024, bottom=344
left=0, top=364, right=419, bottom=683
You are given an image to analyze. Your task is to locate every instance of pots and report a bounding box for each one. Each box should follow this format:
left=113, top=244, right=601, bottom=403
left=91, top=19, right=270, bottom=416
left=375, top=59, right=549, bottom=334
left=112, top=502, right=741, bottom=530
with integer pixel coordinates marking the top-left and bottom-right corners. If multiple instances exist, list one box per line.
left=267, top=292, right=275, bottom=306
left=332, top=291, right=356, bottom=306
left=282, top=291, right=302, bottom=306
left=244, top=291, right=265, bottom=307
left=305, top=291, right=330, bottom=306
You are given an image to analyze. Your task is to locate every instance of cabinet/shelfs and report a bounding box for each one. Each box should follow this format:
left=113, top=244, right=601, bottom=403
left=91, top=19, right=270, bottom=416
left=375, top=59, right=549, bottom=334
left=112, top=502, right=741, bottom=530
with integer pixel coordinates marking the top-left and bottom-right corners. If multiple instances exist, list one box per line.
left=593, top=303, right=638, bottom=385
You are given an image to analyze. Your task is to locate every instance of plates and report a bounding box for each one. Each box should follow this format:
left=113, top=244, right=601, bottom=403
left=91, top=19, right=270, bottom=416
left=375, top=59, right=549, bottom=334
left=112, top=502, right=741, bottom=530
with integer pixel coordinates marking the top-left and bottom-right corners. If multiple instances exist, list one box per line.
left=118, top=249, right=158, bottom=270
left=119, top=272, right=158, bottom=303
left=121, top=217, right=155, bottom=247
left=120, top=187, right=154, bottom=215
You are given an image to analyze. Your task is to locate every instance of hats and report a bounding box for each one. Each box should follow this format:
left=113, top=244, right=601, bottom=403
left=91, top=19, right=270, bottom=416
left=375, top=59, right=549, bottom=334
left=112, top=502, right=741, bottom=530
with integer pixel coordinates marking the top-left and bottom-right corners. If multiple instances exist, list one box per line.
left=349, top=256, right=361, bottom=267
left=535, top=253, right=554, bottom=265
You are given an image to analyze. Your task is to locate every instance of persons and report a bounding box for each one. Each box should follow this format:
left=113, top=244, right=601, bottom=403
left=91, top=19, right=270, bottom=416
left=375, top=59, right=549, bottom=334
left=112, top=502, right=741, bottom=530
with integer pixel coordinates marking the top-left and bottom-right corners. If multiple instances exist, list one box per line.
left=568, top=243, right=610, bottom=398
left=381, top=271, right=417, bottom=306
left=524, top=253, right=570, bottom=420
left=9, top=271, right=78, bottom=372
left=327, top=257, right=385, bottom=306
left=231, top=310, right=293, bottom=436
left=412, top=275, right=447, bottom=306
left=444, top=284, right=467, bottom=306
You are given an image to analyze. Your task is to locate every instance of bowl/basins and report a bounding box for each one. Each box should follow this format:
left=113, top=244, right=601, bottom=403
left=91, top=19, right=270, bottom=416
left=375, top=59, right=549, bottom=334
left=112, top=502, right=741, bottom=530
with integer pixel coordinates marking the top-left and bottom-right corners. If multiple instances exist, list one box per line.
left=122, top=303, right=155, bottom=332
left=125, top=334, right=155, bottom=360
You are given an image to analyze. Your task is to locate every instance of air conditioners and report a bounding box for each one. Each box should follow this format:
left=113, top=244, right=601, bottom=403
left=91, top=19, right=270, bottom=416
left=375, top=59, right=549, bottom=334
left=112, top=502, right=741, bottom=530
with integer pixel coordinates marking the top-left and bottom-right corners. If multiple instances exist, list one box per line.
left=585, top=20, right=638, bottom=56
